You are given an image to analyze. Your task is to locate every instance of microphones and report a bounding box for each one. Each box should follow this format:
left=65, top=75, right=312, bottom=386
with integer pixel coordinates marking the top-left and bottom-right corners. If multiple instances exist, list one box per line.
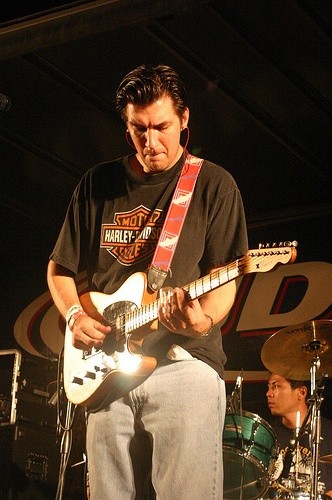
left=226, top=374, right=242, bottom=413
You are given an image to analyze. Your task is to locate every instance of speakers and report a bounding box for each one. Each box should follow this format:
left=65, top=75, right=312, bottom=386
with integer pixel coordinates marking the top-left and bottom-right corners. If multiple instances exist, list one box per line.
left=0, top=349, right=58, bottom=427
left=0, top=422, right=62, bottom=500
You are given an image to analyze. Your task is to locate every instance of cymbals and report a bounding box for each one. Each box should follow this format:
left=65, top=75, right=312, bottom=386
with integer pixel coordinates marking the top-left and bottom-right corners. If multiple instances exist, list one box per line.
left=261, top=320, right=332, bottom=381
left=319, top=454, right=332, bottom=464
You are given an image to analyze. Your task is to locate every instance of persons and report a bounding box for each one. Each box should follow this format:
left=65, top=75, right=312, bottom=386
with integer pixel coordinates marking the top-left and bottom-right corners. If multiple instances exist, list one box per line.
left=259, top=372, right=331, bottom=500
left=49, top=61, right=250, bottom=500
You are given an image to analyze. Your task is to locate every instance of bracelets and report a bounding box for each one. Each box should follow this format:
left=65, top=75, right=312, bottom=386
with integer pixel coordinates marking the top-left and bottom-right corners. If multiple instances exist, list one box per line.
left=68, top=311, right=89, bottom=331
left=202, top=313, right=214, bottom=339
left=66, top=304, right=83, bottom=326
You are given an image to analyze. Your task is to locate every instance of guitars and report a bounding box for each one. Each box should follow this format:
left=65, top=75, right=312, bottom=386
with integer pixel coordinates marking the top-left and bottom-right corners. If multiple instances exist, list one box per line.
left=62, top=239, right=299, bottom=407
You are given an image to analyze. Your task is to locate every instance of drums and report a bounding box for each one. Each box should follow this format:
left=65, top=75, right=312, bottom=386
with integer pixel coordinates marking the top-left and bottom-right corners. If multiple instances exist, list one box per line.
left=221, top=408, right=279, bottom=500
